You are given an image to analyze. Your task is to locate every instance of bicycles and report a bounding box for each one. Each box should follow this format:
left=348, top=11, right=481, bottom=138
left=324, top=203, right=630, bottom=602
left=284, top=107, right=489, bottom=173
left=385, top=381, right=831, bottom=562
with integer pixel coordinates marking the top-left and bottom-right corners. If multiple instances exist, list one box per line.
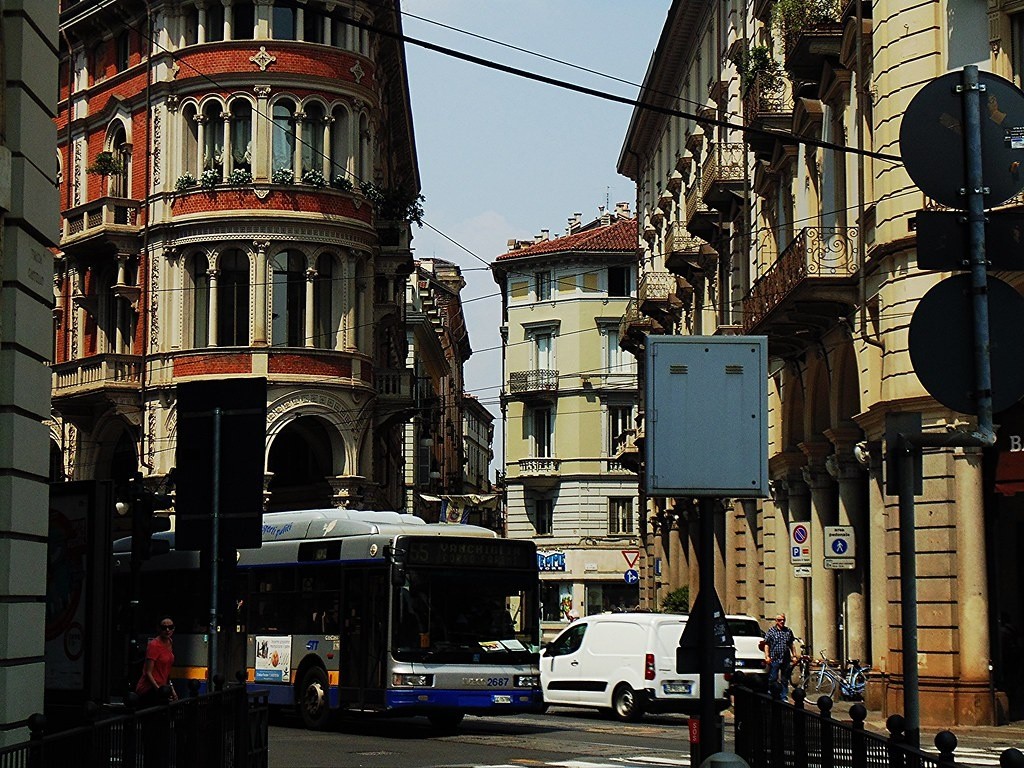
left=789, top=646, right=872, bottom=705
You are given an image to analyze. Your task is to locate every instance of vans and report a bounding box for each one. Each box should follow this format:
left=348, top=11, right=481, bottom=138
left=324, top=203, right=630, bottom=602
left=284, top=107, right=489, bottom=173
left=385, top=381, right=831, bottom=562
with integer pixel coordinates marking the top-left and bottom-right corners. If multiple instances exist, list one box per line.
left=536, top=612, right=731, bottom=723
left=724, top=612, right=771, bottom=692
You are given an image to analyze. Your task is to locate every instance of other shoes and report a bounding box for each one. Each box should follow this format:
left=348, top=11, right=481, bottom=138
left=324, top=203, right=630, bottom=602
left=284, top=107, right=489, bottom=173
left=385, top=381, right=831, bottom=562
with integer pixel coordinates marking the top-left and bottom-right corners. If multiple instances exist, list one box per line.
left=781, top=695, right=788, bottom=701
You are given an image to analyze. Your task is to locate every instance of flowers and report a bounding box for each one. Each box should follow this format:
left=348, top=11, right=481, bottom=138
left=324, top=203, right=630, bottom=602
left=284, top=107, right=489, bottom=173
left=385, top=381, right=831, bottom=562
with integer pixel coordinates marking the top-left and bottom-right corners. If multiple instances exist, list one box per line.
left=271, top=166, right=296, bottom=189
left=200, top=168, right=220, bottom=193
left=359, top=179, right=379, bottom=202
left=301, top=169, right=326, bottom=189
left=331, top=174, right=355, bottom=193
left=174, top=170, right=198, bottom=194
left=225, top=168, right=253, bottom=194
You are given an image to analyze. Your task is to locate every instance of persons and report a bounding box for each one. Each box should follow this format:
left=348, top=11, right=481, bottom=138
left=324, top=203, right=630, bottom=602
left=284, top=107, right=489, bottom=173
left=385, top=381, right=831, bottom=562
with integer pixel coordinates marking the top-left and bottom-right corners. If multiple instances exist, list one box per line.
left=135, top=619, right=179, bottom=702
left=763, top=612, right=797, bottom=704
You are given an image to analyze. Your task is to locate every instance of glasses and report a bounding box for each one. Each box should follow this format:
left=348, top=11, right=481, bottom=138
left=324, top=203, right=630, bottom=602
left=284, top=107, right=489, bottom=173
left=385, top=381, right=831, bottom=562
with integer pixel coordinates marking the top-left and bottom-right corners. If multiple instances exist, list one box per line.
left=161, top=624, right=174, bottom=630
left=777, top=618, right=785, bottom=621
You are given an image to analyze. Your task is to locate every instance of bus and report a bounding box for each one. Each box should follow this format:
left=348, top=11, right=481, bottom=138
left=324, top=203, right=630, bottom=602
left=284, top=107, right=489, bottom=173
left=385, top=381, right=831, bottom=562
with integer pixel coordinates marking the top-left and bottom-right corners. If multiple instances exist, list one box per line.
left=112, top=506, right=544, bottom=733
